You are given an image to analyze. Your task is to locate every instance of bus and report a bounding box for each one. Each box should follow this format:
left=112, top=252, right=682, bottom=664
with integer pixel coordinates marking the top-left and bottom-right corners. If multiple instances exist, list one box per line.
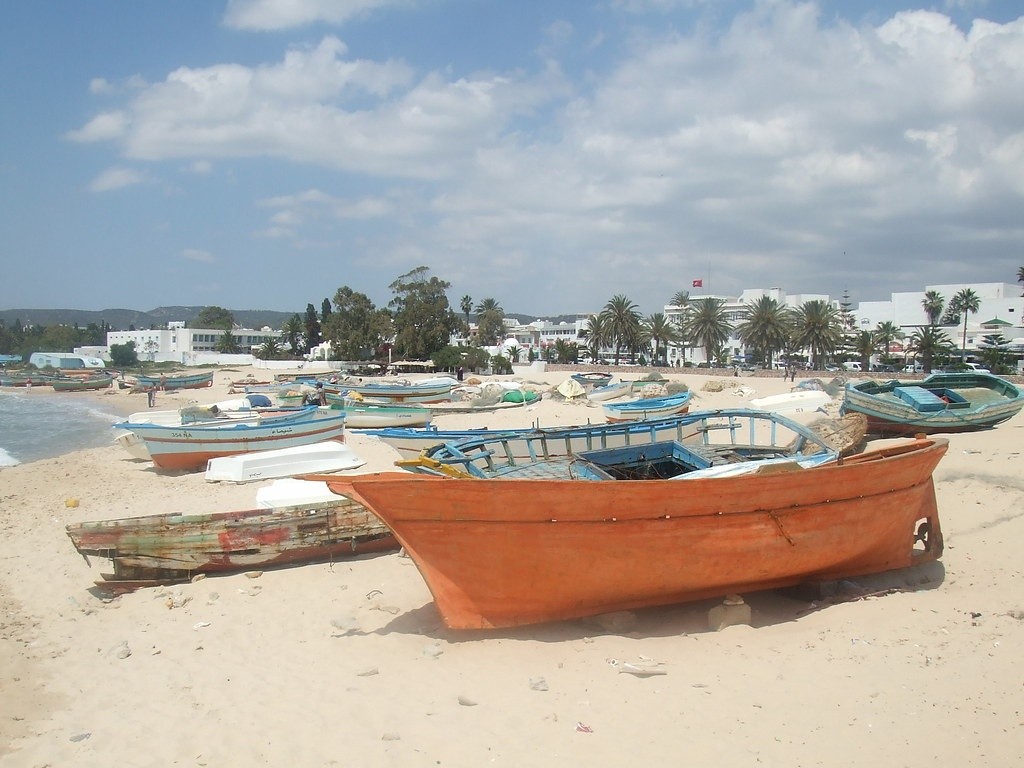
left=597, top=351, right=642, bottom=365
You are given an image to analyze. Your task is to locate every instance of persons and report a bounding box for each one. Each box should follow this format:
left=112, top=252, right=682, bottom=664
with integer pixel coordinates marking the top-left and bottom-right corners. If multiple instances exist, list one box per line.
left=121, top=369, right=125, bottom=379
left=159, top=373, right=166, bottom=392
left=302, top=382, right=326, bottom=406
left=55, top=367, right=60, bottom=376
left=330, top=375, right=339, bottom=384
left=26, top=377, right=32, bottom=390
left=147, top=381, right=157, bottom=408
left=358, top=378, right=364, bottom=386
left=783, top=363, right=796, bottom=382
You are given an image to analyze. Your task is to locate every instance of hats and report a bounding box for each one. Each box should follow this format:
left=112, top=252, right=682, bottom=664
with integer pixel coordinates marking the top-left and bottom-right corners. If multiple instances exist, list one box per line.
left=315, top=382, right=323, bottom=386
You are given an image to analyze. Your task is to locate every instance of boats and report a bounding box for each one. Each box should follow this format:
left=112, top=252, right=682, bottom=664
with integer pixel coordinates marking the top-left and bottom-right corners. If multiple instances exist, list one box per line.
left=601, top=389, right=691, bottom=424
left=229, top=372, right=461, bottom=428
left=572, top=372, right=613, bottom=387
left=112, top=399, right=346, bottom=470
left=620, top=378, right=669, bottom=392
left=0, top=368, right=115, bottom=392
left=587, top=381, right=634, bottom=401
left=204, top=439, right=368, bottom=483
left=838, top=373, right=1024, bottom=436
left=750, top=390, right=832, bottom=411
left=132, top=371, right=214, bottom=390
left=293, top=433, right=950, bottom=632
left=349, top=408, right=841, bottom=479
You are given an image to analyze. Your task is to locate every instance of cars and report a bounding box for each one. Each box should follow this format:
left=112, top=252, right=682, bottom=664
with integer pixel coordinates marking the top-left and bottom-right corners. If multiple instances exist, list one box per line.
left=824, top=362, right=942, bottom=373
left=740, top=360, right=787, bottom=370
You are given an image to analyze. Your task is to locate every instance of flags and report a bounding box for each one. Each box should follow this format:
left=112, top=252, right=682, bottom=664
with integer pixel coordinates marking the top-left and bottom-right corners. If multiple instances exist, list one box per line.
left=693, top=279, right=702, bottom=288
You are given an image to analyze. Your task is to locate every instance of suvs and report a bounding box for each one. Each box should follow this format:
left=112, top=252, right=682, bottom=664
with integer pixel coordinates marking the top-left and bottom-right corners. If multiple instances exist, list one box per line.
left=964, top=363, right=990, bottom=374
